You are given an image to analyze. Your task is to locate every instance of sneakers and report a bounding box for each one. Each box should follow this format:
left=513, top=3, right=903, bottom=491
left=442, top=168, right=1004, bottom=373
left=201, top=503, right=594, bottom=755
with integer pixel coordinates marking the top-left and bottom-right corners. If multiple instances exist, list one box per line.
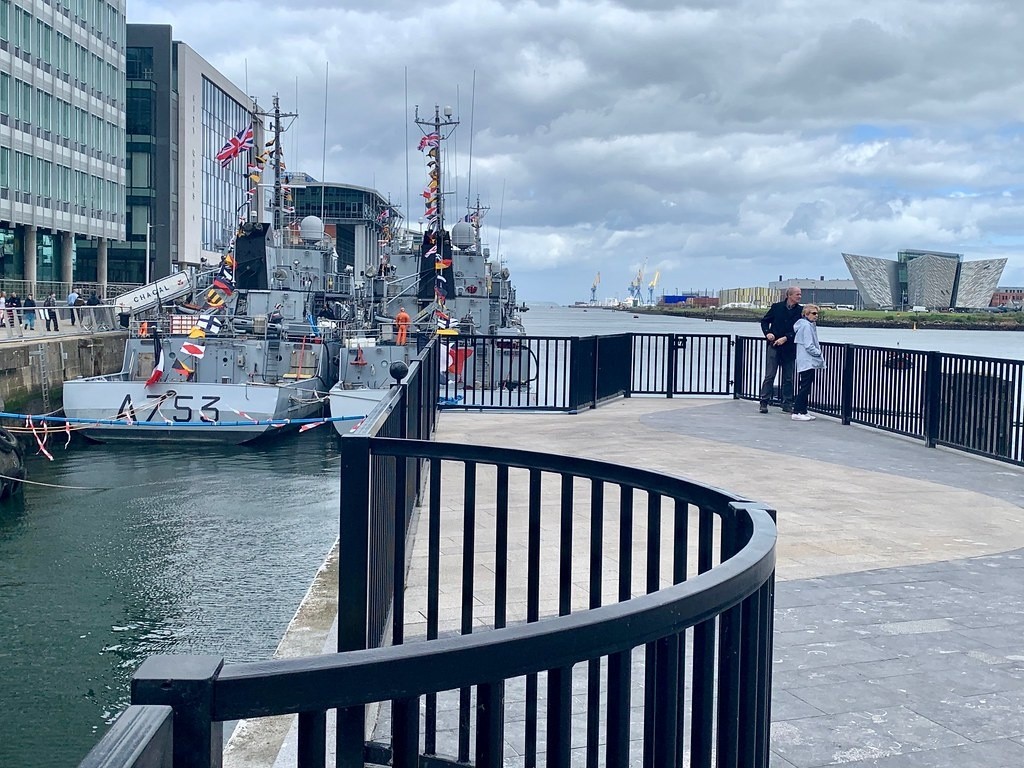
left=792, top=412, right=816, bottom=421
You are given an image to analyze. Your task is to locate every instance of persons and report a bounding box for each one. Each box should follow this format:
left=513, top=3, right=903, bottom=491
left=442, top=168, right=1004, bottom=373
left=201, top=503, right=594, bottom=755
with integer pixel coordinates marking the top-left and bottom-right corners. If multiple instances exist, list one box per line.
left=7, top=291, right=23, bottom=327
left=43, top=292, right=60, bottom=333
left=67, top=288, right=79, bottom=325
left=790, top=304, right=828, bottom=421
left=23, top=294, right=36, bottom=330
left=396, top=307, right=411, bottom=346
left=1, top=290, right=9, bottom=327
left=760, top=287, right=805, bottom=413
left=88, top=290, right=101, bottom=325
left=75, top=294, right=85, bottom=325
left=98, top=295, right=105, bottom=322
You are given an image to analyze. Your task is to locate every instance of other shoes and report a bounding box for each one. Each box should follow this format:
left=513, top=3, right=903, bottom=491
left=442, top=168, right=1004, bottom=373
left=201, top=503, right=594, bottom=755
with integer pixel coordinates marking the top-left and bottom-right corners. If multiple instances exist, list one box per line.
left=782, top=407, right=793, bottom=415
left=759, top=403, right=768, bottom=413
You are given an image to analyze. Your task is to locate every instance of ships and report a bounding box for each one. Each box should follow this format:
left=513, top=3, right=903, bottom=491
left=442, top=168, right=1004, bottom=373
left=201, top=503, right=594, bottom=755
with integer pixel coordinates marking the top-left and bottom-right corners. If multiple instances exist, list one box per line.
left=60, top=64, right=349, bottom=445
left=327, top=65, right=532, bottom=446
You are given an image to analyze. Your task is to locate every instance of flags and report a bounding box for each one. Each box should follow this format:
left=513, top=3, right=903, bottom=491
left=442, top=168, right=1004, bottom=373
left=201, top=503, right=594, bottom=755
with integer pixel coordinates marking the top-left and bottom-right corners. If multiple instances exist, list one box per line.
left=215, top=123, right=253, bottom=168
left=419, top=132, right=459, bottom=332
left=173, top=139, right=300, bottom=380
left=376, top=207, right=392, bottom=272
left=143, top=326, right=164, bottom=388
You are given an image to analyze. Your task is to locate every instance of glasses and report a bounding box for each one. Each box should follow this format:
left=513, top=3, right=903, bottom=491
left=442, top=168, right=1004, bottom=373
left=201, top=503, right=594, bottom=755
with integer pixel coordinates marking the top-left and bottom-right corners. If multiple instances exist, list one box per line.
left=810, top=312, right=818, bottom=315
